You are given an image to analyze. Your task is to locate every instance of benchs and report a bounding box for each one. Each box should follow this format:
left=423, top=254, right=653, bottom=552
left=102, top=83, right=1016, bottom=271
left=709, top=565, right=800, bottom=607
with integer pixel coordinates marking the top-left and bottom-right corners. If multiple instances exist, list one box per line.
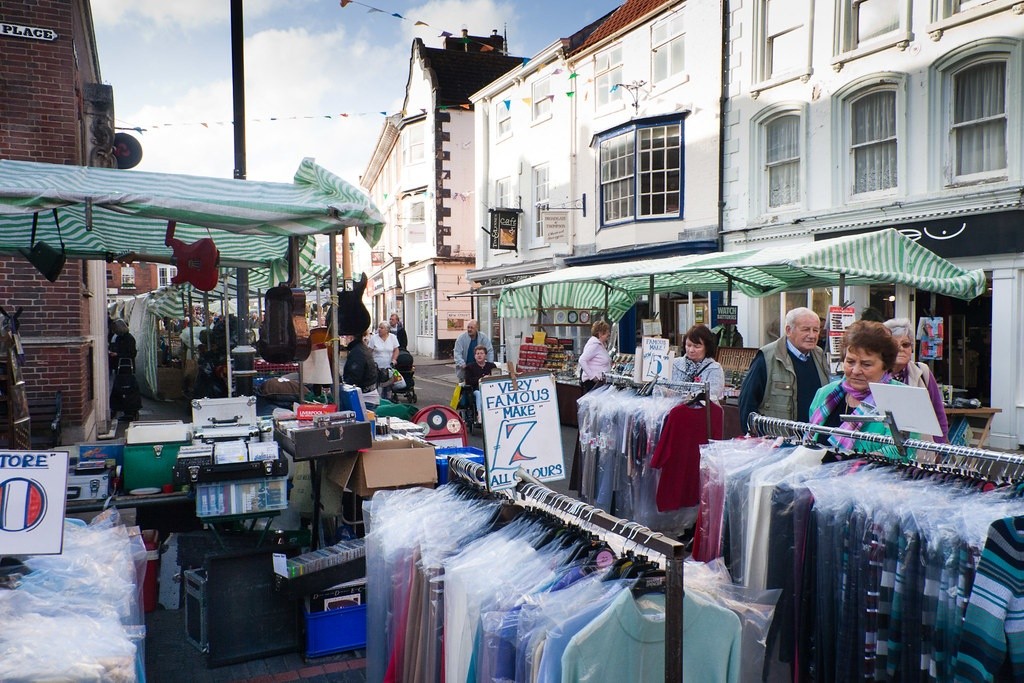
left=0, top=391, right=66, bottom=447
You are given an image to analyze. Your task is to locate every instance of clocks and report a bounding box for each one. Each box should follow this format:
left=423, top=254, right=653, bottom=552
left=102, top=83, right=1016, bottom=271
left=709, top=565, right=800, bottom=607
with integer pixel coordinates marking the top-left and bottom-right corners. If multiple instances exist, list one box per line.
left=554, top=310, right=567, bottom=324
left=578, top=310, right=590, bottom=324
left=568, top=310, right=578, bottom=323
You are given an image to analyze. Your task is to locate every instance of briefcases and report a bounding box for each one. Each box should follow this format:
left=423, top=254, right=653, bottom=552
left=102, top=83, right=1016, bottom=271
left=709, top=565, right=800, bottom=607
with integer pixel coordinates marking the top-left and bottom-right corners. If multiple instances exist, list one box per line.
left=191, top=396, right=258, bottom=438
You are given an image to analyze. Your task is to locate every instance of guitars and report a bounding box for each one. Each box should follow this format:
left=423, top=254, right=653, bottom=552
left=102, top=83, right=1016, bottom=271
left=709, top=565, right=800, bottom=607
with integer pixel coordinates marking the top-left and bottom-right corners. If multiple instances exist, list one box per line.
left=326, top=228, right=371, bottom=335
left=259, top=237, right=312, bottom=363
left=112, top=237, right=219, bottom=291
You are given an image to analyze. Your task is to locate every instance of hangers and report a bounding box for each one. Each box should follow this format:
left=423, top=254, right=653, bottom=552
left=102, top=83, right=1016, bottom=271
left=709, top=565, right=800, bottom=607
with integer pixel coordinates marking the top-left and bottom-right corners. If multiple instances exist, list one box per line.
left=600, top=370, right=712, bottom=406
left=749, top=410, right=1024, bottom=494
left=442, top=453, right=679, bottom=597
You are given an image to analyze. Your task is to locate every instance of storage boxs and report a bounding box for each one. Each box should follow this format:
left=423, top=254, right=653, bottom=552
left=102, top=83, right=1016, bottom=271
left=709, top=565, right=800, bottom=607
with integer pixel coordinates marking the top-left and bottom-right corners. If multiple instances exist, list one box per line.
left=182, top=567, right=209, bottom=652
left=123, top=443, right=191, bottom=486
left=77, top=443, right=123, bottom=462
left=296, top=596, right=368, bottom=657
left=943, top=417, right=974, bottom=466
left=195, top=476, right=289, bottom=517
left=274, top=419, right=373, bottom=460
left=65, top=466, right=116, bottom=501
left=298, top=404, right=338, bottom=420
left=348, top=437, right=435, bottom=499
left=273, top=554, right=365, bottom=599
left=435, top=443, right=486, bottom=482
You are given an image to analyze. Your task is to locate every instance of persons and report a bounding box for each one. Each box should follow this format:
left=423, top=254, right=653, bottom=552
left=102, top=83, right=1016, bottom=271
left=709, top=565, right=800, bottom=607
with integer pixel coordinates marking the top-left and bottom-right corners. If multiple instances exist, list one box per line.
left=669, top=325, right=724, bottom=404
left=389, top=314, right=407, bottom=350
left=464, top=345, right=496, bottom=425
left=878, top=316, right=950, bottom=447
left=368, top=320, right=399, bottom=369
left=738, top=306, right=830, bottom=437
left=454, top=319, right=494, bottom=386
left=577, top=321, right=611, bottom=390
left=808, top=320, right=921, bottom=462
left=110, top=320, right=138, bottom=421
left=162, top=302, right=264, bottom=333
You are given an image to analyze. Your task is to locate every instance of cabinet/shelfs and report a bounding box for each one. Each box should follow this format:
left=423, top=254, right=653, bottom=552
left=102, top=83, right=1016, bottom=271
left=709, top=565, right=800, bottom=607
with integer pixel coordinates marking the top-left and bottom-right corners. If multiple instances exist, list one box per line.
left=0, top=341, right=12, bottom=450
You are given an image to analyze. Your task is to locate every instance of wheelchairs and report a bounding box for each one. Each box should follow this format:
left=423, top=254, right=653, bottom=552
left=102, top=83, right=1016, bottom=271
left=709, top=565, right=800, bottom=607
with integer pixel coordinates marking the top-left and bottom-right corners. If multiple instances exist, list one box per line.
left=458, top=385, right=482, bottom=436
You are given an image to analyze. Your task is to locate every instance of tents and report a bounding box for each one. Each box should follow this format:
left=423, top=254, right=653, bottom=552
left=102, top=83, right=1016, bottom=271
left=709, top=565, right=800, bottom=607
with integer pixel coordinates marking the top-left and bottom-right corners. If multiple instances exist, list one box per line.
left=497, top=229, right=987, bottom=391
left=0, top=156, right=384, bottom=412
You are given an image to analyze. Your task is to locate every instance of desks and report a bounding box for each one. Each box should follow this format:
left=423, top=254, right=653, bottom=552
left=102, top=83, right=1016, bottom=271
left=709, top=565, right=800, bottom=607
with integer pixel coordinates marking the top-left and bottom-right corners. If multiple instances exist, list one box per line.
left=552, top=379, right=587, bottom=430
left=942, top=404, right=1005, bottom=468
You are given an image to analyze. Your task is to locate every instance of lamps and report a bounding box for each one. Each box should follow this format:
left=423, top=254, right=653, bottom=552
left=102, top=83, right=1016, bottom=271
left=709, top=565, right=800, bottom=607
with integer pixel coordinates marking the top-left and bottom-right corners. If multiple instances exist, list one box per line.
left=535, top=193, right=585, bottom=216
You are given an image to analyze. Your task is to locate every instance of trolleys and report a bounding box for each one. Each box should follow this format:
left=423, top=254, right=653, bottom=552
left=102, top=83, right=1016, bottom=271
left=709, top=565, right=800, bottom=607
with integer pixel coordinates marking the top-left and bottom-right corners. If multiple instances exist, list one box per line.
left=374, top=362, right=400, bottom=404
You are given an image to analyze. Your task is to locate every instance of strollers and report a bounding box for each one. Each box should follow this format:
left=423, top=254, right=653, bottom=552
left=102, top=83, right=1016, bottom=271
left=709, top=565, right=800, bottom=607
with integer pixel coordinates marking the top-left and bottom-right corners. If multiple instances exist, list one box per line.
left=391, top=347, right=417, bottom=405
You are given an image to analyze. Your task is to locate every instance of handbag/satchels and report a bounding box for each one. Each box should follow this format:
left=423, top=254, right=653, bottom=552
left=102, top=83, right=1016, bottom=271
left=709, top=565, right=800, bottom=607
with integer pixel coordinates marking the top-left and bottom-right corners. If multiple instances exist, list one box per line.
left=18, top=208, right=66, bottom=283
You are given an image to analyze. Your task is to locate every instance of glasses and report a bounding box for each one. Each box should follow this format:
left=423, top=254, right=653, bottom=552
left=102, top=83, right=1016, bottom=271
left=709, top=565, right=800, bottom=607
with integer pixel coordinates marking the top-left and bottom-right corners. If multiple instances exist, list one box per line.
left=897, top=342, right=912, bottom=348
